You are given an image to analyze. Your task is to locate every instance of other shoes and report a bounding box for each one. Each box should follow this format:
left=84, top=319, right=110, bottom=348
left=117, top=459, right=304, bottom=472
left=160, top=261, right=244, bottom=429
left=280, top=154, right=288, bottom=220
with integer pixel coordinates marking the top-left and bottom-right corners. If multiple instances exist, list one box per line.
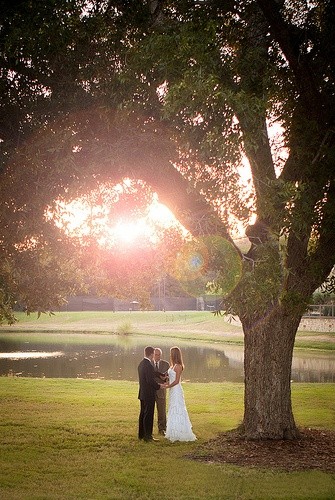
left=144, top=437, right=160, bottom=442
left=159, top=429, right=166, bottom=436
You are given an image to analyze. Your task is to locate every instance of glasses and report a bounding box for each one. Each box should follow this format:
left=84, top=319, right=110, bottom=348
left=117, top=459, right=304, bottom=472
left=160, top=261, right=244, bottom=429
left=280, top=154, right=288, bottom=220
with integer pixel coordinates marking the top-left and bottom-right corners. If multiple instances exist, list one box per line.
left=154, top=354, right=160, bottom=356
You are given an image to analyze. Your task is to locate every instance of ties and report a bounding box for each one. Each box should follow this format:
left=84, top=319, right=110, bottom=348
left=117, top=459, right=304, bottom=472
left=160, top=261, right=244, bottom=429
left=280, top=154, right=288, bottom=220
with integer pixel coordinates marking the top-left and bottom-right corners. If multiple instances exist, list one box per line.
left=155, top=362, right=158, bottom=372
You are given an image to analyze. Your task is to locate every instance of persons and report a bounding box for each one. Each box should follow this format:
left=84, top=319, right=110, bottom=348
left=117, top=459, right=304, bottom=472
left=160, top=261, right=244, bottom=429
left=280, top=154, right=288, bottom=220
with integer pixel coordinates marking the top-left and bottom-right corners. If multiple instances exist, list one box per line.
left=137, top=347, right=197, bottom=442
left=151, top=348, right=170, bottom=436
